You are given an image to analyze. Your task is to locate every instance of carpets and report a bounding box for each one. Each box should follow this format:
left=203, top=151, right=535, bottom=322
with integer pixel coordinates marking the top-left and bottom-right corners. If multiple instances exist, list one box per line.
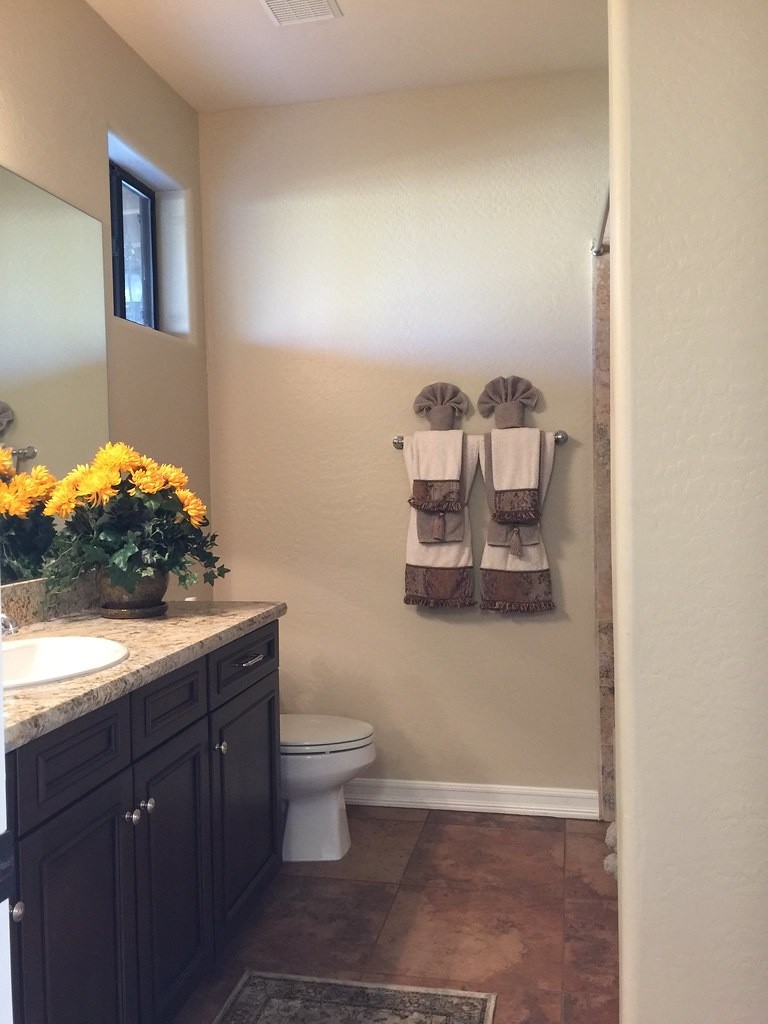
left=209, top=968, right=500, bottom=1024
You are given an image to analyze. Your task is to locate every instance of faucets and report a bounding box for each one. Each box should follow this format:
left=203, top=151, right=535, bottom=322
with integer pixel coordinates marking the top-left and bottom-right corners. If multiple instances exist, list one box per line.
left=0, top=611, right=20, bottom=638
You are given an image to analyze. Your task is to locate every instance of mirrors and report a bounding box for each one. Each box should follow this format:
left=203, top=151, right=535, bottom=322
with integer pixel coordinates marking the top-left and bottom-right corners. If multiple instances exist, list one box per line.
left=1, top=166, right=112, bottom=583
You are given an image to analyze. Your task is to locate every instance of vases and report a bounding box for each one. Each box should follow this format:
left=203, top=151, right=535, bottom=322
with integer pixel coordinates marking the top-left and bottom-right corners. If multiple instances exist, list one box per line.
left=88, top=559, right=173, bottom=620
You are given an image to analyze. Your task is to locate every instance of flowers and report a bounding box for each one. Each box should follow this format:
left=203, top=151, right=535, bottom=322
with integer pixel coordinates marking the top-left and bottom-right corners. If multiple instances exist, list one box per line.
left=38, top=441, right=232, bottom=590
left=0, top=443, right=62, bottom=577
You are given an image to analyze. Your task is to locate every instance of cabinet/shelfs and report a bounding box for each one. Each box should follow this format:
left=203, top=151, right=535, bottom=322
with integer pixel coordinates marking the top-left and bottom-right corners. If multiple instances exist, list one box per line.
left=10, top=620, right=282, bottom=1024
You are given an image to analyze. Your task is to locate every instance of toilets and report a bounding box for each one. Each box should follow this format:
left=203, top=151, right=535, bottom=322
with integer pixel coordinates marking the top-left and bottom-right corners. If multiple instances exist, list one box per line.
left=279, top=711, right=376, bottom=863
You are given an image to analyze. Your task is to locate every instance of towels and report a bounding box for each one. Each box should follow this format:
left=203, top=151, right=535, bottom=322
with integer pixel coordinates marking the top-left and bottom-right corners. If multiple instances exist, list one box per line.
left=403, top=375, right=558, bottom=615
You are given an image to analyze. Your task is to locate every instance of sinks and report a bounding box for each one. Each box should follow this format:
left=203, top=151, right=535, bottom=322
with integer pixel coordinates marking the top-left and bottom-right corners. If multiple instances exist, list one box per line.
left=1, top=635, right=130, bottom=690
